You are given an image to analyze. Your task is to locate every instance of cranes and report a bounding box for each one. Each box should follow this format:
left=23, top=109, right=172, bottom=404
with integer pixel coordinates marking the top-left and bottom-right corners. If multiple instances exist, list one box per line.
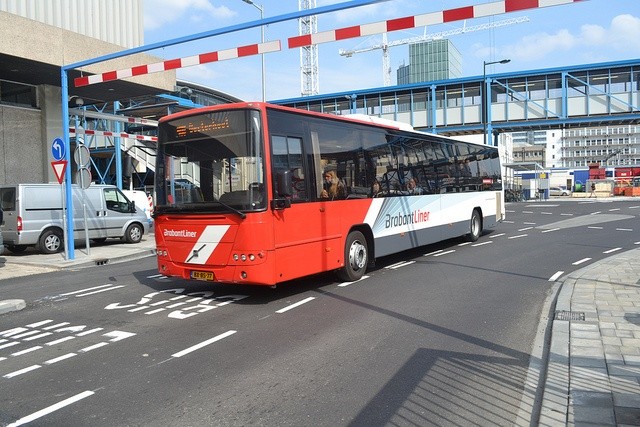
left=338, top=16, right=530, bottom=88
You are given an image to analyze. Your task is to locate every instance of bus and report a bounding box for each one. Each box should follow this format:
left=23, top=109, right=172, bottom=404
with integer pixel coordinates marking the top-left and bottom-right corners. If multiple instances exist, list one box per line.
left=123, top=103, right=505, bottom=288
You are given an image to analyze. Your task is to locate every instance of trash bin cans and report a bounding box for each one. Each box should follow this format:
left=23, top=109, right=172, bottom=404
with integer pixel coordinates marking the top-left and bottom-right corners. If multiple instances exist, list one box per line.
left=539, top=189, right=546, bottom=199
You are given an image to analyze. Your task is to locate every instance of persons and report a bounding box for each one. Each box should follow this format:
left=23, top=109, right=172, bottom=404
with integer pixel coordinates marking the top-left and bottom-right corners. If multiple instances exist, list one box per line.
left=463, top=159, right=478, bottom=177
left=405, top=177, right=423, bottom=195
left=458, top=163, right=467, bottom=176
left=319, top=169, right=347, bottom=200
left=371, top=178, right=385, bottom=197
left=588, top=182, right=597, bottom=198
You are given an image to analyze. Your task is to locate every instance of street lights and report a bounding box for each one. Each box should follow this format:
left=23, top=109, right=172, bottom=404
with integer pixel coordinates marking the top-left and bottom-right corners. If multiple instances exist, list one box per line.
left=242, top=0, right=264, bottom=103
left=483, top=59, right=511, bottom=144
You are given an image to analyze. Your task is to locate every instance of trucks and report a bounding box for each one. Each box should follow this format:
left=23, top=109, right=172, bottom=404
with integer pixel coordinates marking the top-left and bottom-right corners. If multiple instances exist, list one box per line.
left=0, top=184, right=149, bottom=254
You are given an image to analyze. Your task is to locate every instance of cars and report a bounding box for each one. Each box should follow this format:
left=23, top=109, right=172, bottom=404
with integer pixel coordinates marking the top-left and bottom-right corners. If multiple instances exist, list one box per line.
left=535, top=187, right=571, bottom=195
